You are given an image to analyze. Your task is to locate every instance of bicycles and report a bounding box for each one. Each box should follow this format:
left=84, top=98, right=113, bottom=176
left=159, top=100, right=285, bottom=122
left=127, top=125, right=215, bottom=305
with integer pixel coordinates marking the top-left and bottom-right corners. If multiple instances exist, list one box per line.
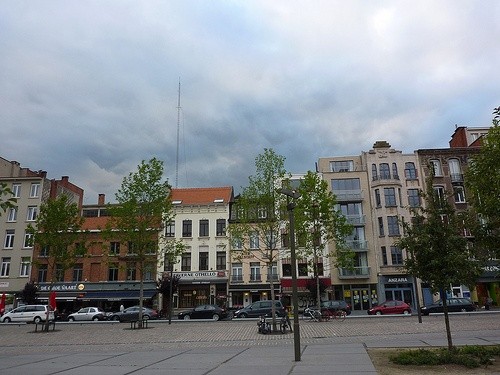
left=302, top=305, right=346, bottom=321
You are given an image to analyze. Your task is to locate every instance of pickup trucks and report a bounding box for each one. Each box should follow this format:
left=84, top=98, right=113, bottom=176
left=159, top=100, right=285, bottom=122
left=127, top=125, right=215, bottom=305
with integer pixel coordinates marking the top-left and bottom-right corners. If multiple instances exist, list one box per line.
left=67, top=307, right=113, bottom=322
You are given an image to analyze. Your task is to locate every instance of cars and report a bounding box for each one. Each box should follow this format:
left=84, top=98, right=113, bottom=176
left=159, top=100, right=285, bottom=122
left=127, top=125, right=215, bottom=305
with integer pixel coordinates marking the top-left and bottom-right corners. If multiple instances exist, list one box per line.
left=419, top=297, right=475, bottom=315
left=235, top=300, right=285, bottom=318
left=178, top=304, right=232, bottom=321
left=1, top=304, right=54, bottom=324
left=303, top=300, right=352, bottom=316
left=112, top=305, right=157, bottom=323
left=368, top=300, right=411, bottom=315
